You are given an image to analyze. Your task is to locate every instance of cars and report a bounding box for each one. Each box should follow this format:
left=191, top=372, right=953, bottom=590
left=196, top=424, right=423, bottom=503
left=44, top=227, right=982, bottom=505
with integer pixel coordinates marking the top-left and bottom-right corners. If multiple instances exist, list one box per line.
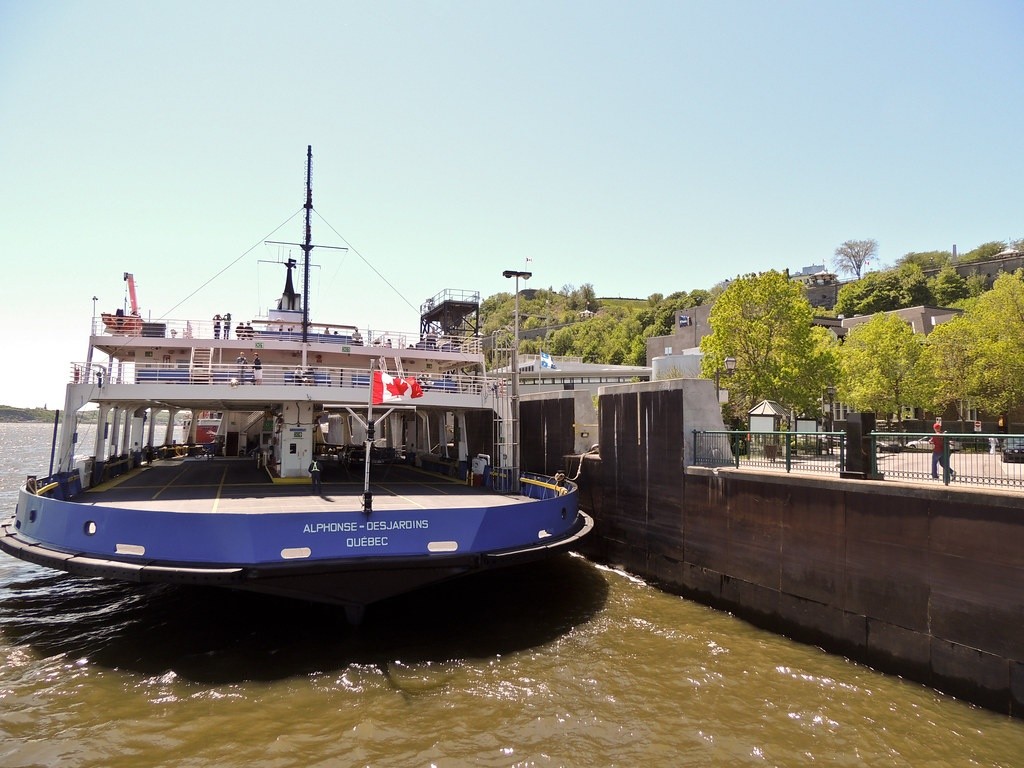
left=906, top=436, right=964, bottom=453
left=1000, top=438, right=1024, bottom=463
left=790, top=435, right=829, bottom=450
left=844, top=436, right=902, bottom=453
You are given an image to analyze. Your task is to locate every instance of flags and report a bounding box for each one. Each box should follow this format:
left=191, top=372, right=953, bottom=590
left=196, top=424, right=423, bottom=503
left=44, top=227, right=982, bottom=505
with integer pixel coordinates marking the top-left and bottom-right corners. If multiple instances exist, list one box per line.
left=371, top=370, right=424, bottom=405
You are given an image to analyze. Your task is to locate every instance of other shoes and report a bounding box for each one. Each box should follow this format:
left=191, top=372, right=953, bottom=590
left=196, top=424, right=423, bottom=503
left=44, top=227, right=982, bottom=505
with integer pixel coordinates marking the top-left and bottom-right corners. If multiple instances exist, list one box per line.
left=951, top=471, right=956, bottom=481
left=928, top=477, right=939, bottom=480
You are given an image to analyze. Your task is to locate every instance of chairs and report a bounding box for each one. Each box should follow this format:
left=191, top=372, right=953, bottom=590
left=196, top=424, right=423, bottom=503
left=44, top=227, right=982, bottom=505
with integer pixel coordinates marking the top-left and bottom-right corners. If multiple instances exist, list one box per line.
left=160, top=445, right=189, bottom=458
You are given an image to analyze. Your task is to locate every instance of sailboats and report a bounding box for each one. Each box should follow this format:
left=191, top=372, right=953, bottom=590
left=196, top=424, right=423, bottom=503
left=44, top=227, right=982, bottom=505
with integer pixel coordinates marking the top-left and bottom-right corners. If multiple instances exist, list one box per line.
left=0, top=145, right=594, bottom=585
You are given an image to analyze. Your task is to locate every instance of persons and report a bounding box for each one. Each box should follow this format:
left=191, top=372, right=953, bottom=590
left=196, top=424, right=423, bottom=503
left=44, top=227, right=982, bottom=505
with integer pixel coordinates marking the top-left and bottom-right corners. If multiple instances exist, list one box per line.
left=213, top=315, right=222, bottom=339
left=988, top=437, right=999, bottom=455
left=222, top=313, right=231, bottom=340
left=294, top=364, right=315, bottom=386
left=235, top=321, right=253, bottom=340
left=352, top=329, right=361, bottom=343
left=929, top=423, right=956, bottom=482
left=132, top=442, right=154, bottom=469
left=251, top=353, right=263, bottom=385
left=237, top=351, right=248, bottom=385
left=738, top=437, right=745, bottom=456
left=324, top=327, right=341, bottom=337
left=410, top=443, right=418, bottom=467
left=308, top=456, right=322, bottom=494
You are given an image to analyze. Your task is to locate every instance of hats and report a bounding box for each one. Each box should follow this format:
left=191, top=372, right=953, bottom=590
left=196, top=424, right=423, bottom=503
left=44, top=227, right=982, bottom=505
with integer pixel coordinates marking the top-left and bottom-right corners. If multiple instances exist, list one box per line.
left=933, top=423, right=941, bottom=429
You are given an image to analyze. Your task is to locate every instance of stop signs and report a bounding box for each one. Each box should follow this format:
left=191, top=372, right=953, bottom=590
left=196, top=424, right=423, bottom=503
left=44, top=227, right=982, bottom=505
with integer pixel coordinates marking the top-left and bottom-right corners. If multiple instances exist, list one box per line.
left=976, top=422, right=981, bottom=427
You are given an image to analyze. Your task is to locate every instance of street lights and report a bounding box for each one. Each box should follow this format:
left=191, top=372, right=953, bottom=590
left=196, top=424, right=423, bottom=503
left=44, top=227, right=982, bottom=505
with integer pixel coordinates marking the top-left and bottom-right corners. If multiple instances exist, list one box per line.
left=826, top=385, right=834, bottom=455
left=716, top=353, right=736, bottom=403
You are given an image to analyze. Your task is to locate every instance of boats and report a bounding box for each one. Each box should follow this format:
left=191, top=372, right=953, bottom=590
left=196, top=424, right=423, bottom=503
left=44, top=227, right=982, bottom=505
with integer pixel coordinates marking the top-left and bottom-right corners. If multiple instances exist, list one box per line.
left=182, top=410, right=222, bottom=444
